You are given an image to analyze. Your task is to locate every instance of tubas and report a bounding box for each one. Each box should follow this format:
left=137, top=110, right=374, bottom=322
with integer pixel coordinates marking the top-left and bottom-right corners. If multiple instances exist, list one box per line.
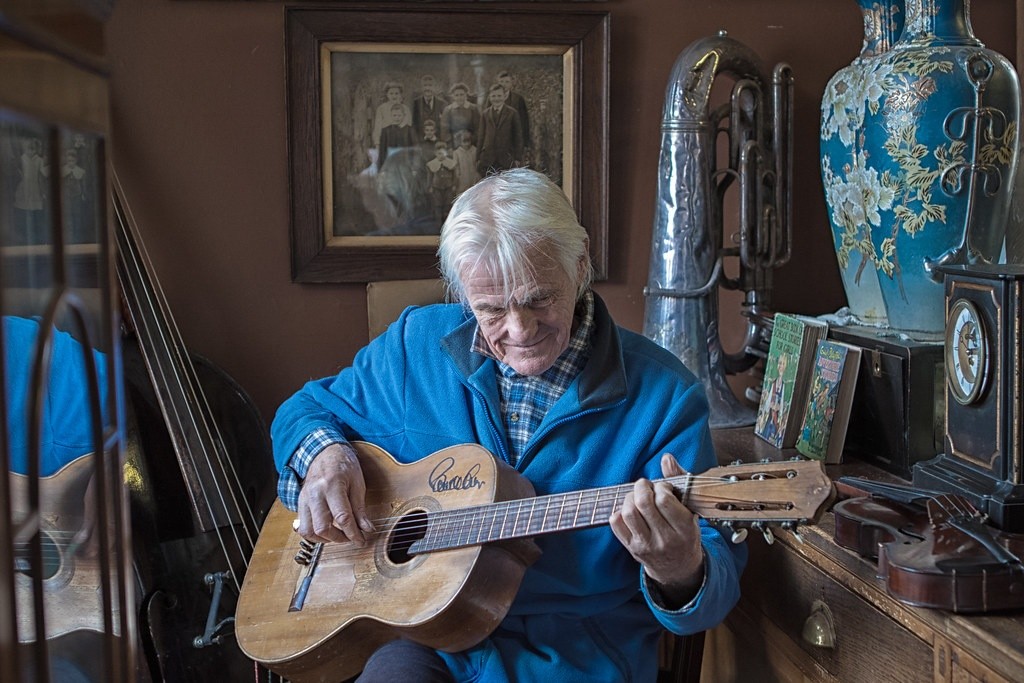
left=641, top=29, right=796, bottom=429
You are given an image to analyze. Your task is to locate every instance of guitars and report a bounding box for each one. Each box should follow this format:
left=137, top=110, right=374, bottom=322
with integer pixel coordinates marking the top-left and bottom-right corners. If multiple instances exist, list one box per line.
left=235, top=440, right=837, bottom=683
left=8, top=450, right=122, bottom=646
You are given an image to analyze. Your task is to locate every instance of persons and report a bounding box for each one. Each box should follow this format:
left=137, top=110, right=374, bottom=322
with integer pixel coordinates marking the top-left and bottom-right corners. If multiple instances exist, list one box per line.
left=364, top=149, right=457, bottom=235
left=376, top=102, right=420, bottom=173
left=411, top=75, right=447, bottom=135
left=422, top=119, right=442, bottom=160
left=2, top=309, right=125, bottom=683
left=376, top=82, right=411, bottom=134
left=483, top=70, right=529, bottom=154
left=267, top=172, right=750, bottom=679
left=478, top=84, right=524, bottom=177
left=426, top=142, right=457, bottom=233
left=452, top=130, right=482, bottom=199
left=441, top=81, right=479, bottom=151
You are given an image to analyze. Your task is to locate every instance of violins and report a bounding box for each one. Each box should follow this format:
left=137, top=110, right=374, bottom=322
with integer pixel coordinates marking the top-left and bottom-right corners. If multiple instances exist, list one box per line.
left=833, top=476, right=1024, bottom=613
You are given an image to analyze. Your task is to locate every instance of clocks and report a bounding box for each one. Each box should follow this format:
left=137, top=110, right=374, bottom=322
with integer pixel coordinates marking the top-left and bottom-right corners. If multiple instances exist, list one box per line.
left=911, top=262, right=1024, bottom=533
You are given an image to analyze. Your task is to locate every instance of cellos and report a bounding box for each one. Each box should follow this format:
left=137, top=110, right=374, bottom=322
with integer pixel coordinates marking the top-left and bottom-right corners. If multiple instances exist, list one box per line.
left=112, top=163, right=291, bottom=683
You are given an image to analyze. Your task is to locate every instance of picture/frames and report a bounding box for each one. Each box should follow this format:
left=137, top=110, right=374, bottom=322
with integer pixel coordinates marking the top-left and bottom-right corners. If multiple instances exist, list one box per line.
left=283, top=5, right=610, bottom=287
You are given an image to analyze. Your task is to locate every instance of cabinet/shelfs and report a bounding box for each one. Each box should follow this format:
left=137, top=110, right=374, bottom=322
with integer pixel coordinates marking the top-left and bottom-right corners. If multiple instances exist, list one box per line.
left=703, top=425, right=1024, bottom=683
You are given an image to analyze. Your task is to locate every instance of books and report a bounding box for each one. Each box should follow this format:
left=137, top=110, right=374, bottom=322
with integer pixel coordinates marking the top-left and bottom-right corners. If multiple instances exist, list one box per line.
left=754, top=312, right=830, bottom=450
left=795, top=339, right=863, bottom=465
